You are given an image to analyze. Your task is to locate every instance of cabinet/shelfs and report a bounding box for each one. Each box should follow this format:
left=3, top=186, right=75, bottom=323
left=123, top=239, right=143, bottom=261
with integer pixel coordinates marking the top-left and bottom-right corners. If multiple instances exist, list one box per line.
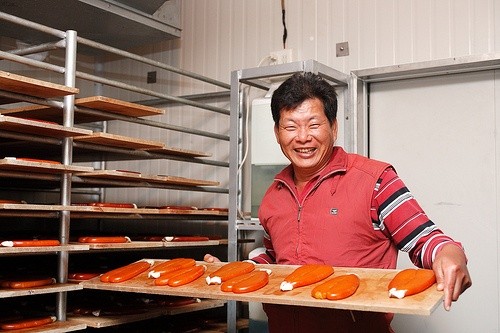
left=0, top=0, right=243, bottom=333
left=228, top=59, right=352, bottom=333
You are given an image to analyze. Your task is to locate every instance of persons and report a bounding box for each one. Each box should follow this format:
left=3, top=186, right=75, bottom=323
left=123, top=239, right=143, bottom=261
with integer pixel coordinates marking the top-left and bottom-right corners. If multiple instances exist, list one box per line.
left=203, top=71, right=472, bottom=333
